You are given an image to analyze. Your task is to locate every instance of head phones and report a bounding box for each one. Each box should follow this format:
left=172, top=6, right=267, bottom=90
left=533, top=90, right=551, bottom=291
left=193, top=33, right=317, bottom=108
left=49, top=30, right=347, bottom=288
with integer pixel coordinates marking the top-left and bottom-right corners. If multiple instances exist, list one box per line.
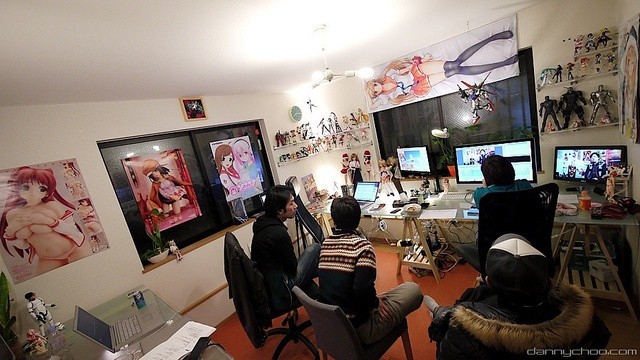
left=618, top=197, right=640, bottom=215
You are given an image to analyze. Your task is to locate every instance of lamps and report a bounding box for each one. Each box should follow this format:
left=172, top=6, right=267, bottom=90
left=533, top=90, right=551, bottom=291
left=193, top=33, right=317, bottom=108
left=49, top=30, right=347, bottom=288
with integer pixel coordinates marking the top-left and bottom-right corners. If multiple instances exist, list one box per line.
left=294, top=49, right=374, bottom=96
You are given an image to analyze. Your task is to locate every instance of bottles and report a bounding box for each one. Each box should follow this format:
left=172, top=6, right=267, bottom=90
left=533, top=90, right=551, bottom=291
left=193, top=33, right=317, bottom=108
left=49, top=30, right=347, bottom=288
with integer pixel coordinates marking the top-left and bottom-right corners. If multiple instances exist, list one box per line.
left=578, top=190, right=591, bottom=210
left=47, top=326, right=73, bottom=360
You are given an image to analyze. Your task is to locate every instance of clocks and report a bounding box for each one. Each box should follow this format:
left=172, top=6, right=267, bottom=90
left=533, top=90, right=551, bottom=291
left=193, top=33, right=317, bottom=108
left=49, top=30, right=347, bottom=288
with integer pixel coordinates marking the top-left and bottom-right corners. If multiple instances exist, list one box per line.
left=290, top=106, right=302, bottom=122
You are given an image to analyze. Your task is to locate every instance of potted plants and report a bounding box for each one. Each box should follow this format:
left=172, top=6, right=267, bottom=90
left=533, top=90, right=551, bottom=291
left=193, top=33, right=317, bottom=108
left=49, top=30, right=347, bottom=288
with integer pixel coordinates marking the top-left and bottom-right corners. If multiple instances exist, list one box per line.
left=139, top=208, right=169, bottom=263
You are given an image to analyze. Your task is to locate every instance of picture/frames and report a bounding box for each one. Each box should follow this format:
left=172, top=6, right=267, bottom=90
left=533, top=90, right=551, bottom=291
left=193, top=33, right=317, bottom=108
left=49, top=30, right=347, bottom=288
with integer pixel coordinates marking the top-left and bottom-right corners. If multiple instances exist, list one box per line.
left=179, top=96, right=208, bottom=122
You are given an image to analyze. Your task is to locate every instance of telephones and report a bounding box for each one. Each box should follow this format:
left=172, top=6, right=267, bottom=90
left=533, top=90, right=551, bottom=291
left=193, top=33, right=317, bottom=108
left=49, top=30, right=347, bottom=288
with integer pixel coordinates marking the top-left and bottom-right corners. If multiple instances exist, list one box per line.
left=590, top=202, right=626, bottom=219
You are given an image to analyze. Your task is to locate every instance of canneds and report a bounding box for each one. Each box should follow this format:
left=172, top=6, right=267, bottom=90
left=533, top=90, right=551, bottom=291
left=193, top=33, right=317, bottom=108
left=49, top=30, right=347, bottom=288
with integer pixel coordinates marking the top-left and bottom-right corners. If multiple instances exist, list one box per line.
left=133, top=291, right=146, bottom=310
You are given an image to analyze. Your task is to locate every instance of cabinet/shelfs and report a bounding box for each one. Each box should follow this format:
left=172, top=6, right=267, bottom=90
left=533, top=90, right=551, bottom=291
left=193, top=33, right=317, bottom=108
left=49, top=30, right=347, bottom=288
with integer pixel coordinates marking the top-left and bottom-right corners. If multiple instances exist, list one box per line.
left=275, top=136, right=322, bottom=166
left=539, top=44, right=619, bottom=133
left=323, top=126, right=373, bottom=154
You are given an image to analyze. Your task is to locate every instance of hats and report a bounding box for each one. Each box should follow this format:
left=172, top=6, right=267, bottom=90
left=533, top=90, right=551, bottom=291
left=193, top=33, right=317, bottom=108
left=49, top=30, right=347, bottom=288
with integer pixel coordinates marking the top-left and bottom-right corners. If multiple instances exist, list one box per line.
left=485, top=233, right=548, bottom=292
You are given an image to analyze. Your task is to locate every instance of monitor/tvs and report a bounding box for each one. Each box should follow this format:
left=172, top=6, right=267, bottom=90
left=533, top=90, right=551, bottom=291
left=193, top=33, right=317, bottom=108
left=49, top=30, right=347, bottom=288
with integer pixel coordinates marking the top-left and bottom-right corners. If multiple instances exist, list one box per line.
left=553, top=146, right=628, bottom=196
left=395, top=145, right=433, bottom=176
left=452, top=137, right=537, bottom=184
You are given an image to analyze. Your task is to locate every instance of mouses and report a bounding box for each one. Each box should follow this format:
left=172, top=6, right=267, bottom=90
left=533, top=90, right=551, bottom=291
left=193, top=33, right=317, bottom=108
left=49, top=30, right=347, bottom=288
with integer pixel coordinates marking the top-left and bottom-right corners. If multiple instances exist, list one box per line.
left=373, top=204, right=380, bottom=209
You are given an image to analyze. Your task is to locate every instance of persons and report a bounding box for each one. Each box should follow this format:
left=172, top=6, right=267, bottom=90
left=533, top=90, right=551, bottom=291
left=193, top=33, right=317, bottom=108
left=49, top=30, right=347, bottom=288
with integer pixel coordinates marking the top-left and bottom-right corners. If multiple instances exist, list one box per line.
left=410, top=176, right=450, bottom=197
left=407, top=153, right=416, bottom=169
left=602, top=163, right=633, bottom=207
left=318, top=195, right=425, bottom=345
left=539, top=96, right=562, bottom=132
left=537, top=68, right=556, bottom=92
left=25, top=328, right=49, bottom=356
left=570, top=114, right=582, bottom=128
left=378, top=160, right=394, bottom=196
left=551, top=64, right=563, bottom=83
left=456, top=71, right=495, bottom=125
left=456, top=155, right=534, bottom=273
left=596, top=111, right=610, bottom=125
left=545, top=120, right=556, bottom=132
left=588, top=85, right=616, bottom=125
left=464, top=150, right=494, bottom=164
left=275, top=99, right=374, bottom=198
left=558, top=87, right=587, bottom=129
left=595, top=27, right=612, bottom=50
left=603, top=51, right=617, bottom=72
left=585, top=32, right=597, bottom=52
left=422, top=233, right=612, bottom=360
left=168, top=239, right=183, bottom=261
left=251, top=185, right=321, bottom=310
left=567, top=62, right=575, bottom=80
left=561, top=151, right=606, bottom=179
left=574, top=34, right=586, bottom=53
left=593, top=53, right=603, bottom=73
left=579, top=57, right=590, bottom=77
left=25, top=292, right=65, bottom=339
left=385, top=156, right=406, bottom=195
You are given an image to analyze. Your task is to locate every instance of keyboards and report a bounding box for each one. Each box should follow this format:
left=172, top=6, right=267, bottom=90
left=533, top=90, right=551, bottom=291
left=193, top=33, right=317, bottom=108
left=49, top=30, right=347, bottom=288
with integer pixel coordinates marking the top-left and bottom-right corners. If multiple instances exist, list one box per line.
left=437, top=193, right=473, bottom=203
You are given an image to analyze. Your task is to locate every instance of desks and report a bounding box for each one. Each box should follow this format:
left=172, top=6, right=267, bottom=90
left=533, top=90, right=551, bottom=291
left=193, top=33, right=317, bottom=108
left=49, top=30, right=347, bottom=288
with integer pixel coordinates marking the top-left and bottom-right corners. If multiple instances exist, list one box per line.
left=306, top=188, right=639, bottom=320
left=22, top=284, right=233, bottom=360
left=458, top=184, right=559, bottom=278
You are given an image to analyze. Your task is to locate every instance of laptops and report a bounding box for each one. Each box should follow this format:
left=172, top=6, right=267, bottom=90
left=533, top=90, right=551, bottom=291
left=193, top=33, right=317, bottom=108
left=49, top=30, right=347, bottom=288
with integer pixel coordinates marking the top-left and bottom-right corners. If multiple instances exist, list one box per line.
left=73, top=290, right=166, bottom=353
left=353, top=181, right=379, bottom=209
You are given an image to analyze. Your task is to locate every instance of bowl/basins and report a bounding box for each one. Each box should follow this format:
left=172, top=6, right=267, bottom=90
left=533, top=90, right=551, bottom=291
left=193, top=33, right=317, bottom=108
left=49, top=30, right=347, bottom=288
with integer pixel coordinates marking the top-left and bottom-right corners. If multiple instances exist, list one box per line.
left=403, top=204, right=421, bottom=215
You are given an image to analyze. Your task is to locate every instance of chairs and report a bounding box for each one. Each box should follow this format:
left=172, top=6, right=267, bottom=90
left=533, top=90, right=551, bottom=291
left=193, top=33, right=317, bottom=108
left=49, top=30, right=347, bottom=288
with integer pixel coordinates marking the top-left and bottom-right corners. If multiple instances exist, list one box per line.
left=224, top=233, right=320, bottom=360
left=293, top=287, right=414, bottom=360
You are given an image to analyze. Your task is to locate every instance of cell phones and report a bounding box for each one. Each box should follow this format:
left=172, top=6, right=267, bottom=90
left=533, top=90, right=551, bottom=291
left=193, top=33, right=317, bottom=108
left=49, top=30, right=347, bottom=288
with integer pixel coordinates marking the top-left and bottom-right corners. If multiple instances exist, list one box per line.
left=127, top=285, right=150, bottom=299
left=390, top=208, right=402, bottom=213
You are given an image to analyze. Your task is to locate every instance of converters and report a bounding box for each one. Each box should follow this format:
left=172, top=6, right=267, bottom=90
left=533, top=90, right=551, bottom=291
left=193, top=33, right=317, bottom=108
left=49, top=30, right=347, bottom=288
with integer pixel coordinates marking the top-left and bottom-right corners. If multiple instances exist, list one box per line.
left=421, top=249, right=433, bottom=256
left=432, top=242, right=441, bottom=250
